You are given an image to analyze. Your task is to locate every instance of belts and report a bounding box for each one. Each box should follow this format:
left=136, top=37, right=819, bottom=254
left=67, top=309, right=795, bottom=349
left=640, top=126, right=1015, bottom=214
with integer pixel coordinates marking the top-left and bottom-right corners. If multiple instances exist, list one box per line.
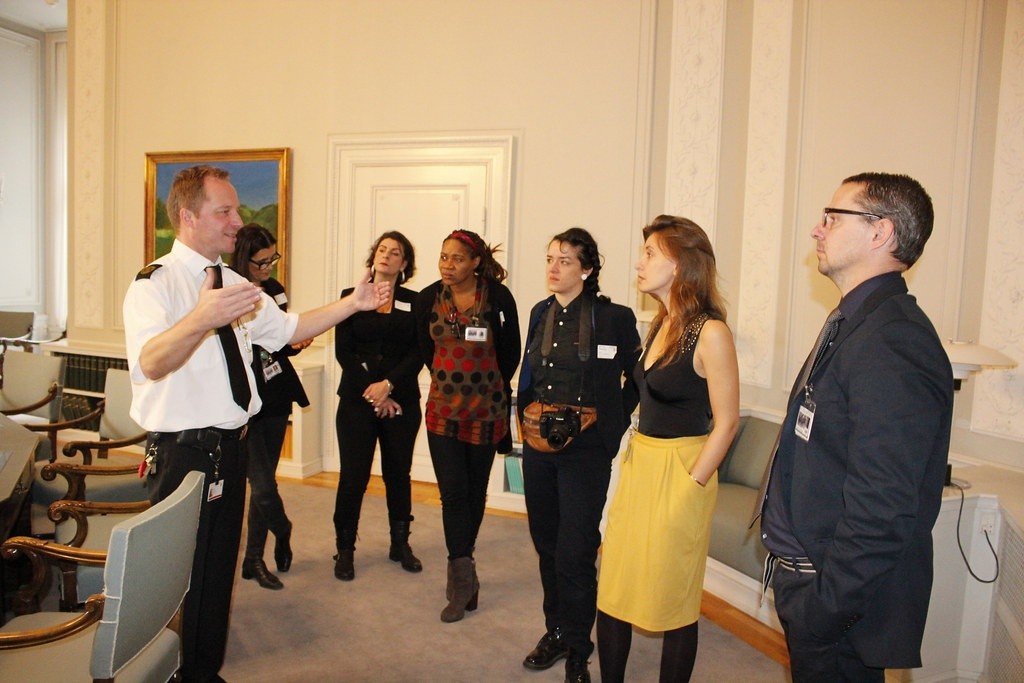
left=160, top=425, right=249, bottom=440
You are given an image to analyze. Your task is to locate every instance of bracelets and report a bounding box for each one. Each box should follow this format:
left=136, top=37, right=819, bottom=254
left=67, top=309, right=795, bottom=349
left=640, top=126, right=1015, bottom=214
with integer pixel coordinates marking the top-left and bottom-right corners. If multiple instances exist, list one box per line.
left=386, top=380, right=392, bottom=394
left=689, top=472, right=705, bottom=487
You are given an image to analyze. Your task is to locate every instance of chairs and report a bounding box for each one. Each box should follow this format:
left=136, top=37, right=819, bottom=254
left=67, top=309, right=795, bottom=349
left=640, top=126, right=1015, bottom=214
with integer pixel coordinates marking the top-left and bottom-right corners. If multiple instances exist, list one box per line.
left=0, top=308, right=206, bottom=683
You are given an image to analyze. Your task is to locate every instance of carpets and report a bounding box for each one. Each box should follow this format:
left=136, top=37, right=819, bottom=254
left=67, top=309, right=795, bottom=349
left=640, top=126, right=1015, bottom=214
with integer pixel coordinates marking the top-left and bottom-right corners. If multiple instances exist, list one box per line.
left=0, top=449, right=792, bottom=683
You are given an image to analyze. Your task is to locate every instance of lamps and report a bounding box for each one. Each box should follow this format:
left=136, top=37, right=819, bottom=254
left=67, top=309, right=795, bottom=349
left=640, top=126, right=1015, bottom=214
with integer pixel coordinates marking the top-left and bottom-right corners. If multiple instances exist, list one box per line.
left=941, top=339, right=1018, bottom=487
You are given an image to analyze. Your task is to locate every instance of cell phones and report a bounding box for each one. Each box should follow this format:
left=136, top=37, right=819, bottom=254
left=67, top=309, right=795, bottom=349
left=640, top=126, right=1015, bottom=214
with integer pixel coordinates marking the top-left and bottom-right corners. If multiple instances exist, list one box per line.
left=374, top=407, right=400, bottom=415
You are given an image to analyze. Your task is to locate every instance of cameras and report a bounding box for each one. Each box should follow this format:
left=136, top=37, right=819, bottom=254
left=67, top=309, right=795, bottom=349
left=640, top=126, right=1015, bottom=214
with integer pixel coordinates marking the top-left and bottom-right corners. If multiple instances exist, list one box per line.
left=539, top=406, right=580, bottom=449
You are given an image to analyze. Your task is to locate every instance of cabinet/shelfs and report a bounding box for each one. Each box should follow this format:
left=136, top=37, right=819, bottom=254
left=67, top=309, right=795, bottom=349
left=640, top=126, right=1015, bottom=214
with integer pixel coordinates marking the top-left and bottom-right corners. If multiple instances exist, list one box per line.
left=39, top=335, right=324, bottom=480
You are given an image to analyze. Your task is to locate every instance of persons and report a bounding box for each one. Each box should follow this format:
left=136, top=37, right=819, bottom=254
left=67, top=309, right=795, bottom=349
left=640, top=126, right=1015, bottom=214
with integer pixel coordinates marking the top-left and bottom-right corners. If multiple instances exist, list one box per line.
left=412, top=228, right=521, bottom=622
left=758, top=171, right=954, bottom=683
left=332, top=229, right=424, bottom=581
left=516, top=227, right=645, bottom=683
left=122, top=166, right=391, bottom=683
left=210, top=489, right=214, bottom=497
left=227, top=222, right=315, bottom=591
left=594, top=214, right=739, bottom=683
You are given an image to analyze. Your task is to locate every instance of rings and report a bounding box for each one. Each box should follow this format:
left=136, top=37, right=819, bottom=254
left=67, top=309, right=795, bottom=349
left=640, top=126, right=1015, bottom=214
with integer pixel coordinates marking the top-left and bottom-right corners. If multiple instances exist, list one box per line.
left=370, top=400, right=374, bottom=403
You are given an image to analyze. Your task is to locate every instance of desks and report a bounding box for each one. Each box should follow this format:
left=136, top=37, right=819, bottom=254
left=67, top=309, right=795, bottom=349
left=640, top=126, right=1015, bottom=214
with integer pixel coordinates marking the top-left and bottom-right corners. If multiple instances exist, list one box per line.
left=0, top=413, right=42, bottom=620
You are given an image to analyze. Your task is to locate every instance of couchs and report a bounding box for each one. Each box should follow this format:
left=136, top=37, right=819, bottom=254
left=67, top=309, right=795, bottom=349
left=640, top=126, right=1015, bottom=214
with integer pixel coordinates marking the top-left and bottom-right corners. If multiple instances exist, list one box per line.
left=709, top=414, right=795, bottom=636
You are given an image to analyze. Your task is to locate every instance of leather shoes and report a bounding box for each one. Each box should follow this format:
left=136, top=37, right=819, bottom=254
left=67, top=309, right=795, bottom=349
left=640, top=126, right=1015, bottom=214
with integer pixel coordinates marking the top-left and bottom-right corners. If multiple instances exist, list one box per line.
left=274, top=521, right=292, bottom=572
left=565, top=653, right=591, bottom=683
left=242, top=557, right=283, bottom=590
left=523, top=624, right=570, bottom=671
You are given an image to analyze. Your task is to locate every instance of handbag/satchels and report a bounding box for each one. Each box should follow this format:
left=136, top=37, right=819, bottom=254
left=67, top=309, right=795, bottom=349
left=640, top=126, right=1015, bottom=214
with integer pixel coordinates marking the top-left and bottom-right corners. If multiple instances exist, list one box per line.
left=521, top=401, right=597, bottom=453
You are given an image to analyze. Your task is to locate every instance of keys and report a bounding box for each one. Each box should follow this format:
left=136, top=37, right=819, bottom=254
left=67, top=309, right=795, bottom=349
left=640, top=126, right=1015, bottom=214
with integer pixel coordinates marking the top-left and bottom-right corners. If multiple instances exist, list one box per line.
left=141, top=454, right=156, bottom=476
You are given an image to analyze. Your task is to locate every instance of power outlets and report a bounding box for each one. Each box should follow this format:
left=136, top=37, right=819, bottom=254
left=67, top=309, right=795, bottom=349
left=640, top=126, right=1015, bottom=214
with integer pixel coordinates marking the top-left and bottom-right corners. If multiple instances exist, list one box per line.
left=981, top=513, right=994, bottom=535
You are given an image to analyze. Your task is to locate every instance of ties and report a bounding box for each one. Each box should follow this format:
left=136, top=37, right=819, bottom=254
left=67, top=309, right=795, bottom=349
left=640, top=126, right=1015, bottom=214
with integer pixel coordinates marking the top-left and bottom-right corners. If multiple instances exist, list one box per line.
left=205, top=263, right=252, bottom=413
left=790, top=307, right=844, bottom=406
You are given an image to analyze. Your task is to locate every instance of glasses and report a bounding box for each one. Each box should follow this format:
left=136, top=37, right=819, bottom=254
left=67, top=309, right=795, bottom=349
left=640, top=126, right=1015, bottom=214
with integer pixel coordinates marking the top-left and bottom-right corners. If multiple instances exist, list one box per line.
left=250, top=251, right=281, bottom=271
left=821, top=207, right=882, bottom=228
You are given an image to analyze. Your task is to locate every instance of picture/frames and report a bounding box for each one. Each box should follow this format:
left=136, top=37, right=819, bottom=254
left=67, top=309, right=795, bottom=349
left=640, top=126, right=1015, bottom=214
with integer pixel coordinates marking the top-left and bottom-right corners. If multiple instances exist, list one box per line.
left=144, top=148, right=291, bottom=299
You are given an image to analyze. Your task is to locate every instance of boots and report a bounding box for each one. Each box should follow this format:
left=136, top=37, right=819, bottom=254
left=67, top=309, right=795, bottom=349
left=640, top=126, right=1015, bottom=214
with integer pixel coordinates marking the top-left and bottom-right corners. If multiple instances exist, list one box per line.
left=388, top=518, right=423, bottom=573
left=332, top=518, right=358, bottom=581
left=441, top=556, right=480, bottom=623
